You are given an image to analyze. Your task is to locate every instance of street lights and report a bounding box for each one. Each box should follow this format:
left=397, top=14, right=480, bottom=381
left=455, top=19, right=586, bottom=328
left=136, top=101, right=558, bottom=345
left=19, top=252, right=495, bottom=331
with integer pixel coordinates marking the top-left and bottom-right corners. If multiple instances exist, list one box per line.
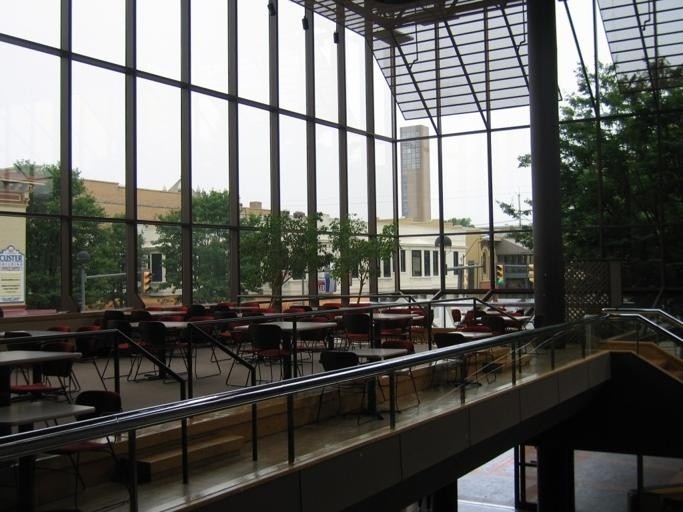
left=459, top=236, right=489, bottom=290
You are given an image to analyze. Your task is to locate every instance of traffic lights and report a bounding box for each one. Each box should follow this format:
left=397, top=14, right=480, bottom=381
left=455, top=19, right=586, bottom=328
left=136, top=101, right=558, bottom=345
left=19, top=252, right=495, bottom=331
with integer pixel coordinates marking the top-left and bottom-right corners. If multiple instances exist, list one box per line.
left=527, top=264, right=534, bottom=285
left=141, top=269, right=152, bottom=293
left=496, top=264, right=503, bottom=278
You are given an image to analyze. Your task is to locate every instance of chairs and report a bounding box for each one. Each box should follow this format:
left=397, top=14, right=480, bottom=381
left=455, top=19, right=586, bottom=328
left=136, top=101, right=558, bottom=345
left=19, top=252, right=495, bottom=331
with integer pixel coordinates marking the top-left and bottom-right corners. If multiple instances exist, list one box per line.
left=0, top=301, right=535, bottom=512
